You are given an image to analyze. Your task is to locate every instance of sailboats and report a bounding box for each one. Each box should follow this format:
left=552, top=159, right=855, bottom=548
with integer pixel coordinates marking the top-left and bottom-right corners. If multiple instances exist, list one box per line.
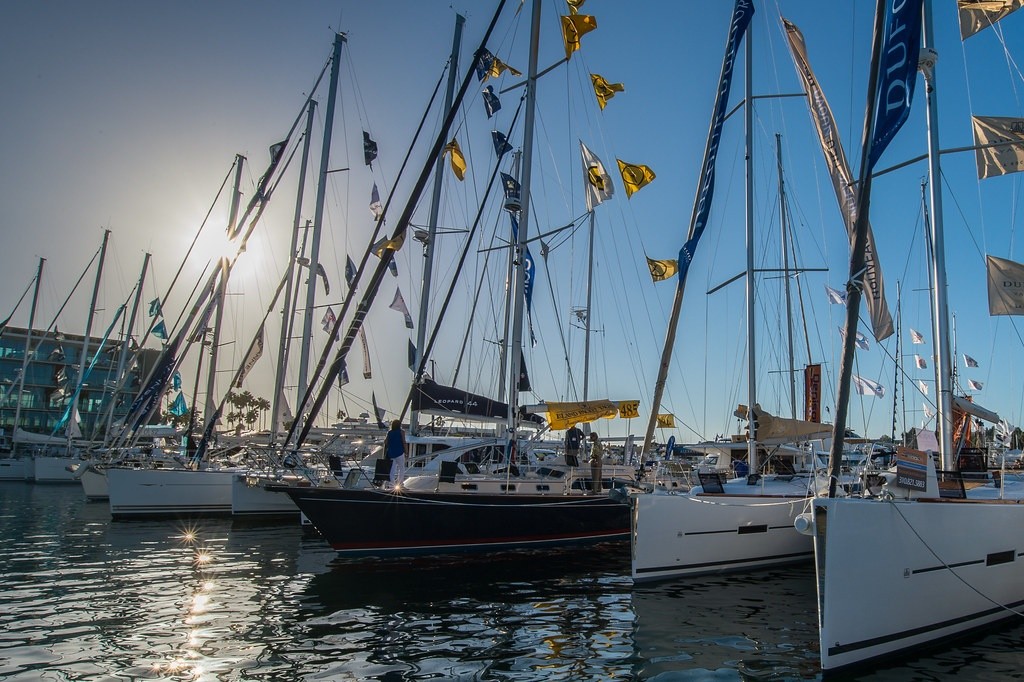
left=2, top=2, right=1023, bottom=588
left=792, top=0, right=1024, bottom=681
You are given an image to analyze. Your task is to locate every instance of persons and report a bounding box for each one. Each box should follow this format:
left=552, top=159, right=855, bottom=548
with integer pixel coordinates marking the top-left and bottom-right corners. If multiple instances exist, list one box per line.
left=588, top=432, right=603, bottom=494
left=384, top=420, right=410, bottom=490
left=564, top=426, right=585, bottom=495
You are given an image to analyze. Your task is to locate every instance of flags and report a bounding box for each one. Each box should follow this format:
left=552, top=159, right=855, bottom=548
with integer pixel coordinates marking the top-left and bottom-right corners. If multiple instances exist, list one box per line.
left=969, top=380, right=983, bottom=391
left=958, top=0, right=1024, bottom=41
left=825, top=284, right=886, bottom=399
left=148, top=296, right=189, bottom=417
left=963, top=354, right=978, bottom=367
left=566, top=0, right=678, bottom=283
left=47, top=325, right=74, bottom=407
left=317, top=254, right=359, bottom=386
left=130, top=337, right=138, bottom=350
left=619, top=400, right=639, bottom=418
left=656, top=414, right=674, bottom=428
left=473, top=44, right=523, bottom=199
left=443, top=138, right=467, bottom=182
left=910, top=328, right=935, bottom=430
left=362, top=131, right=417, bottom=375
left=972, top=116, right=1024, bottom=180
left=987, top=255, right=1024, bottom=316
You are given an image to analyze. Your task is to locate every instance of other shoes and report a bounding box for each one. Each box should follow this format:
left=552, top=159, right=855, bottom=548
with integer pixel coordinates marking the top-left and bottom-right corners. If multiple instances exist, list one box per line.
left=589, top=491, right=598, bottom=495
